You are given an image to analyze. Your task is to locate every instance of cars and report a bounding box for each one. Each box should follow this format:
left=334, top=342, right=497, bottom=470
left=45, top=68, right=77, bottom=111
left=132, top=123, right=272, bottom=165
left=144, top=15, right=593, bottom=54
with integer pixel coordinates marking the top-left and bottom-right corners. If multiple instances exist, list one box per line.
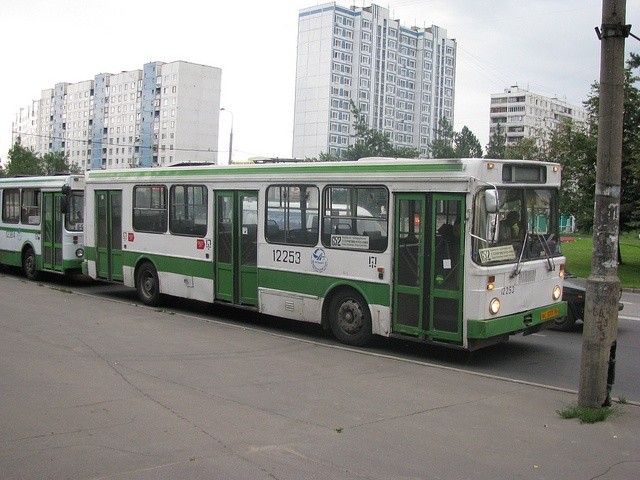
left=545, top=268, right=623, bottom=331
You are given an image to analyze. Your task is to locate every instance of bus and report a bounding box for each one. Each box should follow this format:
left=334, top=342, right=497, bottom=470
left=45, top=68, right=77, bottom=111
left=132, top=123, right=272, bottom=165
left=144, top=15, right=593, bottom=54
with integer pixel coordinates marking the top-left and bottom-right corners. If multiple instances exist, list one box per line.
left=82, top=154, right=568, bottom=349
left=0, top=172, right=84, bottom=280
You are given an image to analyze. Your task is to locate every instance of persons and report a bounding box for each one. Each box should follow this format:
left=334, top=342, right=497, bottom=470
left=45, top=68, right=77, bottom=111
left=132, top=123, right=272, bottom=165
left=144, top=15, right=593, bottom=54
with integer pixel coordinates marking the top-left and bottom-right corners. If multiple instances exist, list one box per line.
left=76, top=211, right=84, bottom=222
left=441, top=217, right=462, bottom=264
left=16, top=209, right=27, bottom=222
left=28, top=209, right=35, bottom=216
left=499, top=212, right=525, bottom=244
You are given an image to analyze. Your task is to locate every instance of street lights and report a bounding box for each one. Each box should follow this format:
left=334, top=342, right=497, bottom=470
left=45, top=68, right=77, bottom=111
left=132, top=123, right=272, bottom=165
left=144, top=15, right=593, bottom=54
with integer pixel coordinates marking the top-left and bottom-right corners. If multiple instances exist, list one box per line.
left=219, top=108, right=234, bottom=164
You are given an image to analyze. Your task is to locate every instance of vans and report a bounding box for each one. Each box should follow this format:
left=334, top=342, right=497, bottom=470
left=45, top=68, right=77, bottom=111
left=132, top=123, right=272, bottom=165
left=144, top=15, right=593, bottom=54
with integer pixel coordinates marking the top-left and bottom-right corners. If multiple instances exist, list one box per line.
left=224, top=201, right=389, bottom=237
